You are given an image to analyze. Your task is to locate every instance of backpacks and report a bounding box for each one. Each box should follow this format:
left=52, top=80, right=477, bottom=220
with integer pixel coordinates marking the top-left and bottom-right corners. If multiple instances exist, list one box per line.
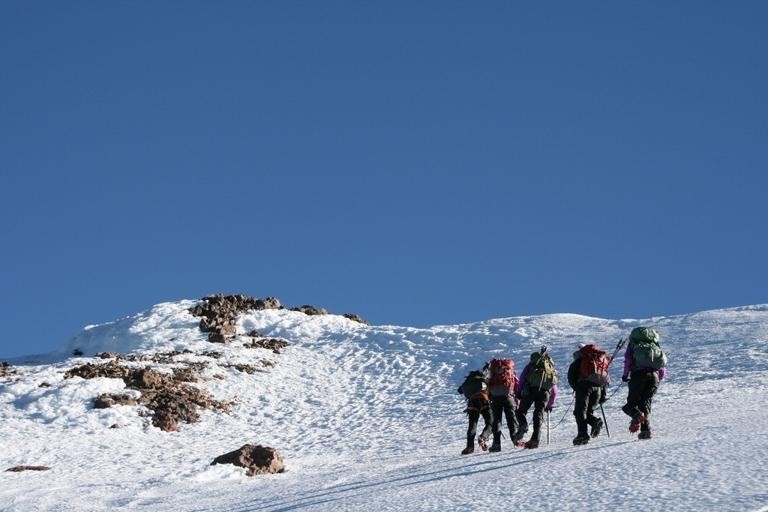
left=579, top=344, right=610, bottom=385
left=462, top=371, right=488, bottom=397
left=630, top=327, right=667, bottom=369
left=488, top=360, right=515, bottom=397
left=526, top=346, right=558, bottom=391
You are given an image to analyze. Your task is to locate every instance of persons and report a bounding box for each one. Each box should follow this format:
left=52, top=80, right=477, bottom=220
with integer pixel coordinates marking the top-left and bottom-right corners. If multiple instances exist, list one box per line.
left=457, top=369, right=494, bottom=455
left=566, top=343, right=610, bottom=446
left=514, top=351, right=558, bottom=449
left=621, top=327, right=667, bottom=439
left=482, top=356, right=527, bottom=452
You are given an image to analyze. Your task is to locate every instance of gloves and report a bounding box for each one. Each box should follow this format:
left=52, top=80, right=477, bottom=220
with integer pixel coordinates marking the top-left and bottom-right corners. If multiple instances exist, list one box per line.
left=622, top=376, right=631, bottom=382
left=545, top=408, right=552, bottom=413
left=516, top=392, right=521, bottom=400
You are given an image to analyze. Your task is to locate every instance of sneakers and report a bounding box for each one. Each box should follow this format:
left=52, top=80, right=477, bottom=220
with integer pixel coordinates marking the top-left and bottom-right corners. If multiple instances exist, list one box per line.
left=639, top=431, right=650, bottom=439
left=573, top=436, right=589, bottom=444
left=629, top=412, right=644, bottom=432
left=462, top=427, right=538, bottom=453
left=591, top=419, right=603, bottom=438
